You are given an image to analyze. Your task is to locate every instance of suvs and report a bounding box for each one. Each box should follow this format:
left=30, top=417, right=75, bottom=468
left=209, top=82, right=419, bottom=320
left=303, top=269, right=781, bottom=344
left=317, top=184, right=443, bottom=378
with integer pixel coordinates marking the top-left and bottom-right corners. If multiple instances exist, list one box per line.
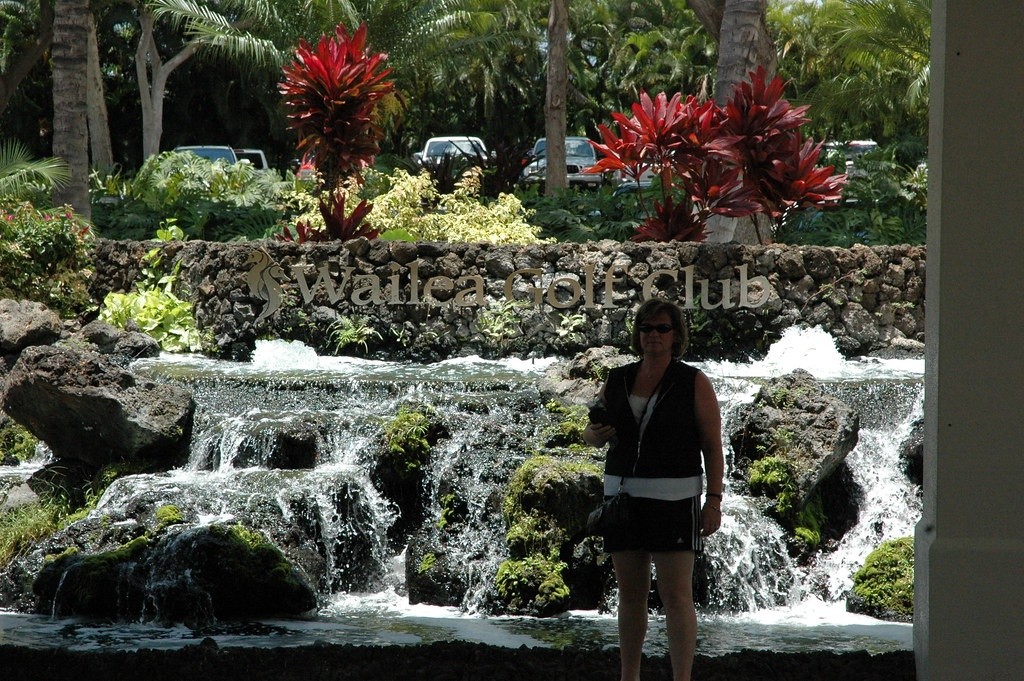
left=166, top=142, right=237, bottom=170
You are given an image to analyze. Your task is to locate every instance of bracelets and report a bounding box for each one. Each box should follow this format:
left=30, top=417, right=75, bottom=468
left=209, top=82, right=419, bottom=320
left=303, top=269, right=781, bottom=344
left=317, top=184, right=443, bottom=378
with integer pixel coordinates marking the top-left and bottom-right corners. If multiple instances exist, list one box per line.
left=704, top=502, right=722, bottom=514
left=704, top=491, right=722, bottom=501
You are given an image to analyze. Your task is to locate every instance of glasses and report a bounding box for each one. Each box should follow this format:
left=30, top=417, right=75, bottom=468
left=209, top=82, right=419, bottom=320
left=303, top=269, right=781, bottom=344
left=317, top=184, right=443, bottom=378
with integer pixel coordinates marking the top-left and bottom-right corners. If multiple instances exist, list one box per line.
left=639, top=323, right=672, bottom=333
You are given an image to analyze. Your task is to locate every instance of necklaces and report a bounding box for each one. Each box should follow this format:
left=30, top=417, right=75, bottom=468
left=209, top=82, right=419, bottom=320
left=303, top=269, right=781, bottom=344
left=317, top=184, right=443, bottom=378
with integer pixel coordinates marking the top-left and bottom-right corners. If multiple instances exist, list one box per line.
left=640, top=365, right=667, bottom=379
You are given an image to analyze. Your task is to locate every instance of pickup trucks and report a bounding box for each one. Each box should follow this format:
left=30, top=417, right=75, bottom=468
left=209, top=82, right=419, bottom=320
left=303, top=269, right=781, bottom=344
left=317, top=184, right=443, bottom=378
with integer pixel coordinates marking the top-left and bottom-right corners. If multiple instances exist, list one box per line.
left=611, top=163, right=676, bottom=189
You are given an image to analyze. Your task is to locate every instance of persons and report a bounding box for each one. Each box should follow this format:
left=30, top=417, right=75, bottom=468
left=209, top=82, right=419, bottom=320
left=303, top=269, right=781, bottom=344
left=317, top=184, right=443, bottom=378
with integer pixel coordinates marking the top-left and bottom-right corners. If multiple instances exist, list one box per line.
left=583, top=297, right=724, bottom=681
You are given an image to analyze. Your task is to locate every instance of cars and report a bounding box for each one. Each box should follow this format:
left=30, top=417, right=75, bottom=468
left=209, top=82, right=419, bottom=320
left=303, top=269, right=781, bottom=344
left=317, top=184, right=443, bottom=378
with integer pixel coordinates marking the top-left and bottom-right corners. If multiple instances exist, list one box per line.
left=412, top=135, right=498, bottom=169
left=816, top=140, right=878, bottom=168
left=233, top=148, right=269, bottom=170
left=291, top=150, right=374, bottom=191
left=522, top=135, right=601, bottom=190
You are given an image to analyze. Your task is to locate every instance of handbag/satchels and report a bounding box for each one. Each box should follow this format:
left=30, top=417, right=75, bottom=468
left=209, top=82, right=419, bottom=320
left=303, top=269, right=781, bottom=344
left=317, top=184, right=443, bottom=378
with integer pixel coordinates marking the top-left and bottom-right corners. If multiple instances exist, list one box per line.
left=587, top=493, right=628, bottom=536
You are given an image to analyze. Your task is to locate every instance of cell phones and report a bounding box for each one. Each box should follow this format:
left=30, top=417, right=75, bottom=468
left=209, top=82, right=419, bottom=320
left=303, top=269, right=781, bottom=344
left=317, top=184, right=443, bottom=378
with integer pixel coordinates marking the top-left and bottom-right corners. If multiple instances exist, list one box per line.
left=588, top=408, right=612, bottom=431
